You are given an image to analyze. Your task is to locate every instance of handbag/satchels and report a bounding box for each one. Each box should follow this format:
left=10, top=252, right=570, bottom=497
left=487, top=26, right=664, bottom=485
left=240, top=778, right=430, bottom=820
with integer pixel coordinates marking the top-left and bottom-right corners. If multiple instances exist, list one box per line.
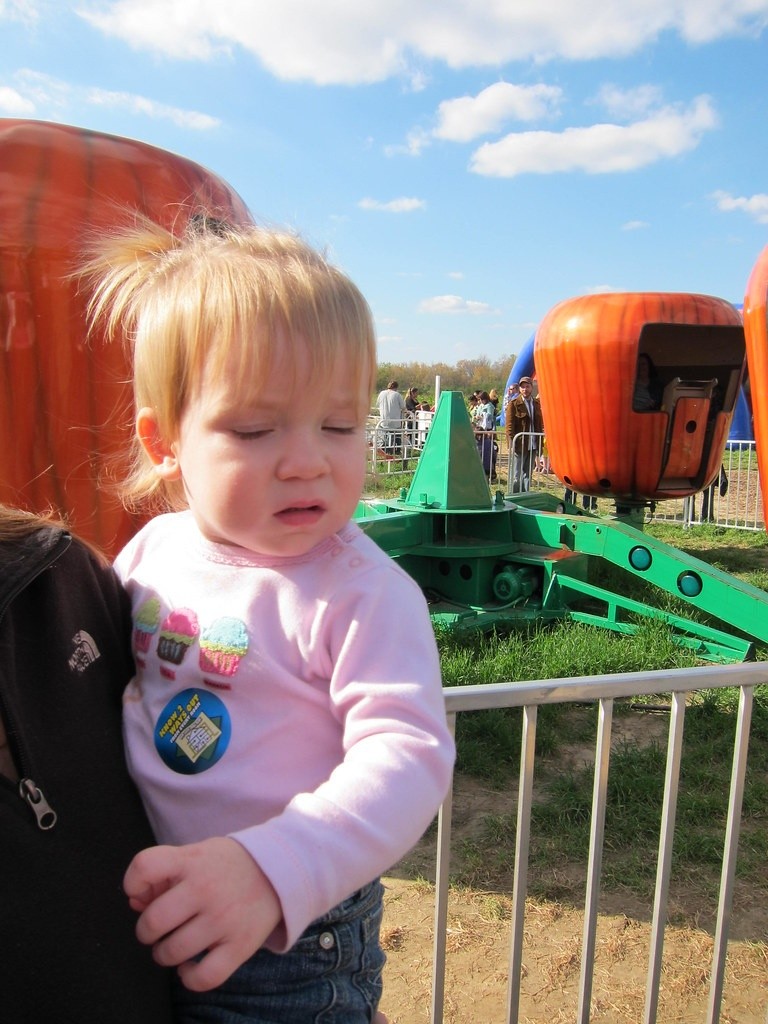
left=474, top=426, right=487, bottom=441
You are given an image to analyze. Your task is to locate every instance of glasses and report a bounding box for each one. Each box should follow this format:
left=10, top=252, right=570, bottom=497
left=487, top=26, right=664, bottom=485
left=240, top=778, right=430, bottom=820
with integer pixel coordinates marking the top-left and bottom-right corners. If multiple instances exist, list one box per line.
left=509, top=387, right=516, bottom=390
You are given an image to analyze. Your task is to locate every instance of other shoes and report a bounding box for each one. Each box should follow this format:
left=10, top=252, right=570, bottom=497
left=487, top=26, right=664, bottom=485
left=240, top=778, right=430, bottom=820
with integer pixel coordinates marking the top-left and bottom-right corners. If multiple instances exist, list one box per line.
left=535, top=465, right=553, bottom=473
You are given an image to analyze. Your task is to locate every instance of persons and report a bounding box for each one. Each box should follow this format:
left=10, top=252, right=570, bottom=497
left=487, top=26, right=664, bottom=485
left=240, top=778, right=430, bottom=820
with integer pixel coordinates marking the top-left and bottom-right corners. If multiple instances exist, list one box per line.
left=375, top=376, right=552, bottom=494
left=0, top=497, right=164, bottom=1023
left=105, top=232, right=459, bottom=1023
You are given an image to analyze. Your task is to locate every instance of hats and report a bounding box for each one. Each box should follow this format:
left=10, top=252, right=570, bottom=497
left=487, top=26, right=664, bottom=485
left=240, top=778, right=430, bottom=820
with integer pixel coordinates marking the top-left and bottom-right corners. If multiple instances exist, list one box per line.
left=518, top=376, right=532, bottom=386
left=477, top=392, right=489, bottom=399
left=468, top=395, right=478, bottom=402
